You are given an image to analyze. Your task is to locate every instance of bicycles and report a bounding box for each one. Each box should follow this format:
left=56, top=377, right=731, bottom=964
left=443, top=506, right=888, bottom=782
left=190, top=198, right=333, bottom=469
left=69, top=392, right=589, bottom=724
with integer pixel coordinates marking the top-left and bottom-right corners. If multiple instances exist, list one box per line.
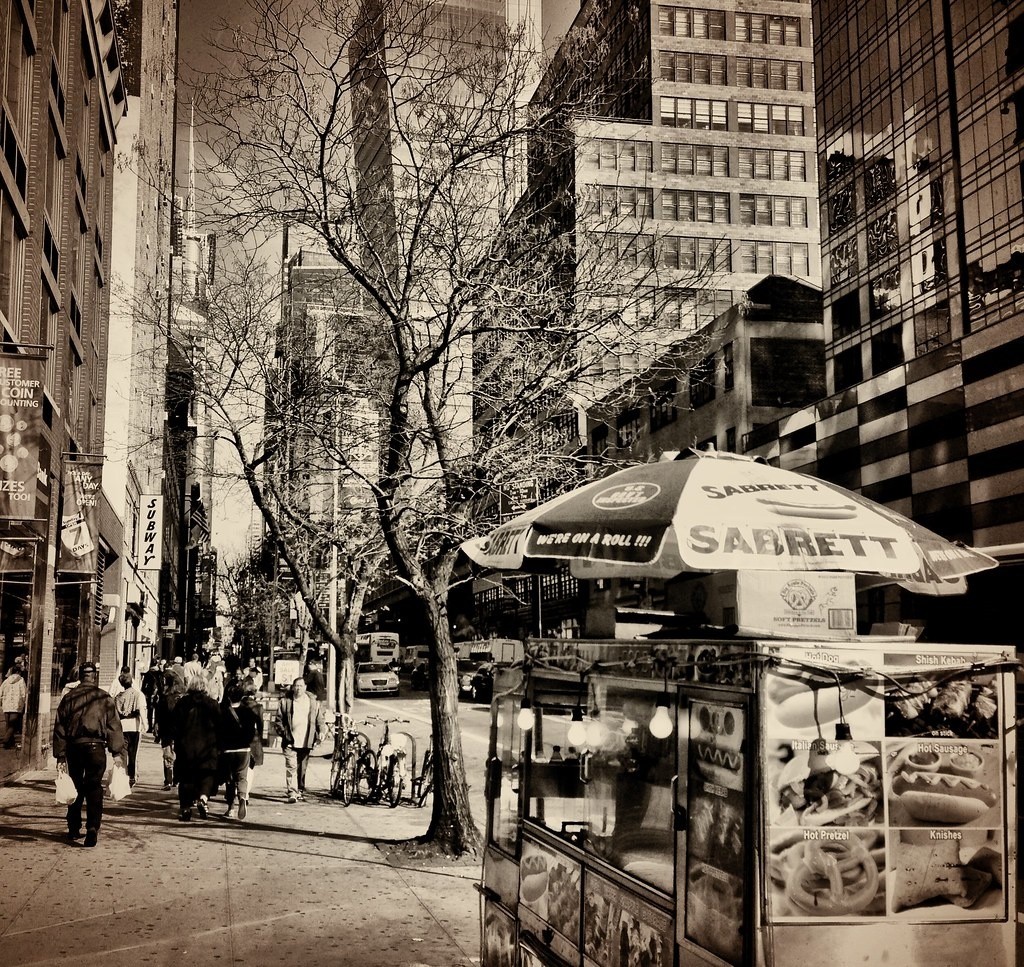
left=328, top=714, right=436, bottom=808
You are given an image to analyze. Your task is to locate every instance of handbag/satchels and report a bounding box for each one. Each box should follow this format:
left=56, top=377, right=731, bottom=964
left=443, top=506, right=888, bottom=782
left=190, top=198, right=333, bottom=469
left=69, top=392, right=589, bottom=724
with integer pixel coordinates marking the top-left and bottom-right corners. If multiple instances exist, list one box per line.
left=250, top=736, right=263, bottom=765
left=109, top=764, right=132, bottom=801
left=55, top=770, right=78, bottom=804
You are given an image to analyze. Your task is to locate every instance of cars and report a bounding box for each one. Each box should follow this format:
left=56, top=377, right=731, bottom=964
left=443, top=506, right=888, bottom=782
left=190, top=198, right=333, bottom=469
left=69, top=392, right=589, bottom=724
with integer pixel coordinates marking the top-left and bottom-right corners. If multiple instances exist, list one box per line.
left=455, top=657, right=479, bottom=701
left=411, top=660, right=432, bottom=692
left=470, top=662, right=496, bottom=702
left=352, top=661, right=401, bottom=699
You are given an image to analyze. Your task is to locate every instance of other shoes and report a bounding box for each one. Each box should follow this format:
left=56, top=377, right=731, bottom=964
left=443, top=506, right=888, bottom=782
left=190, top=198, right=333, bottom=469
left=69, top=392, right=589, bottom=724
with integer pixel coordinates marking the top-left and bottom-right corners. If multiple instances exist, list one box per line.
left=288, top=795, right=296, bottom=802
left=130, top=779, right=135, bottom=788
left=69, top=828, right=79, bottom=838
left=161, top=783, right=171, bottom=790
left=197, top=801, right=208, bottom=819
left=238, top=799, right=246, bottom=819
left=85, top=827, right=97, bottom=847
left=179, top=809, right=192, bottom=821
left=297, top=793, right=303, bottom=799
left=172, top=782, right=176, bottom=787
left=225, top=810, right=233, bottom=817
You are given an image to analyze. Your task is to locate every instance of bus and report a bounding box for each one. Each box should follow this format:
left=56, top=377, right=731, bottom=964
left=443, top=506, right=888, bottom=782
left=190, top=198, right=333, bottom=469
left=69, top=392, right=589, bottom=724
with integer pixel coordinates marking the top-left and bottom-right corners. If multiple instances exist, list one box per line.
left=357, top=632, right=402, bottom=670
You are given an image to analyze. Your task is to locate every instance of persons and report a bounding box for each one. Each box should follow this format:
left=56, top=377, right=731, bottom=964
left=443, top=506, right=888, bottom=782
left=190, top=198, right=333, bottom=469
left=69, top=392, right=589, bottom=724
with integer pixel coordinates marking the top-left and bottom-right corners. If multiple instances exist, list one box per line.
left=54, top=662, right=125, bottom=847
left=0, top=667, right=27, bottom=750
left=141, top=653, right=263, bottom=821
left=153, top=671, right=181, bottom=791
left=273, top=677, right=326, bottom=803
left=389, top=657, right=402, bottom=675
left=115, top=674, right=149, bottom=788
left=61, top=667, right=81, bottom=701
left=5, top=654, right=28, bottom=687
left=108, top=665, right=140, bottom=697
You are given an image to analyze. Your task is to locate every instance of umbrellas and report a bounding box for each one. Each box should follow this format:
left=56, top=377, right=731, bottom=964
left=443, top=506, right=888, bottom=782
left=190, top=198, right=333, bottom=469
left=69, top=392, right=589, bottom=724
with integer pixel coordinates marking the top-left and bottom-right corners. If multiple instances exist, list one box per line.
left=458, top=440, right=1000, bottom=598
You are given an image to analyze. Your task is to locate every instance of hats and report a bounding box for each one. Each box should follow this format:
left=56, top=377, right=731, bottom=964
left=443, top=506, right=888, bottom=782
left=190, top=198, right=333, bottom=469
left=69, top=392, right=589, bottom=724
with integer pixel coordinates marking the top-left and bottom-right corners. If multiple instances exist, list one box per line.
left=249, top=667, right=260, bottom=673
left=174, top=657, right=182, bottom=663
left=79, top=662, right=96, bottom=673
left=210, top=656, right=220, bottom=662
